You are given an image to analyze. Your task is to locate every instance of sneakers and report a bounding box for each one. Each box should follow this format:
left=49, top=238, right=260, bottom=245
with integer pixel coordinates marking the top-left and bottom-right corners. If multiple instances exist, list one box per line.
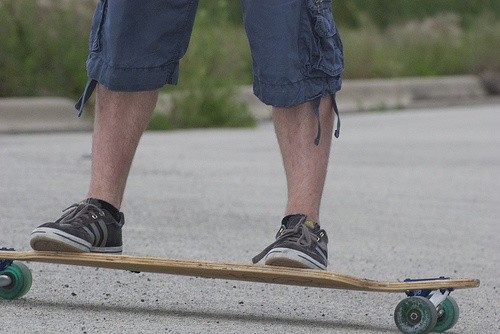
left=30, top=201, right=125, bottom=253
left=252, top=215, right=328, bottom=270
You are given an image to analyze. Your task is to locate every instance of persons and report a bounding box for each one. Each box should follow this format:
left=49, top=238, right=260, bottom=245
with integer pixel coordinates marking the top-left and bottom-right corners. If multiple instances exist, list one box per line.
left=29, top=0, right=345, bottom=272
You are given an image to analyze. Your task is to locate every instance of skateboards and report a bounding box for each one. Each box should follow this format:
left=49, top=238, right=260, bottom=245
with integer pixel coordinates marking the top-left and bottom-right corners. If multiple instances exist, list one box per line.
left=0, top=247, right=480, bottom=334
